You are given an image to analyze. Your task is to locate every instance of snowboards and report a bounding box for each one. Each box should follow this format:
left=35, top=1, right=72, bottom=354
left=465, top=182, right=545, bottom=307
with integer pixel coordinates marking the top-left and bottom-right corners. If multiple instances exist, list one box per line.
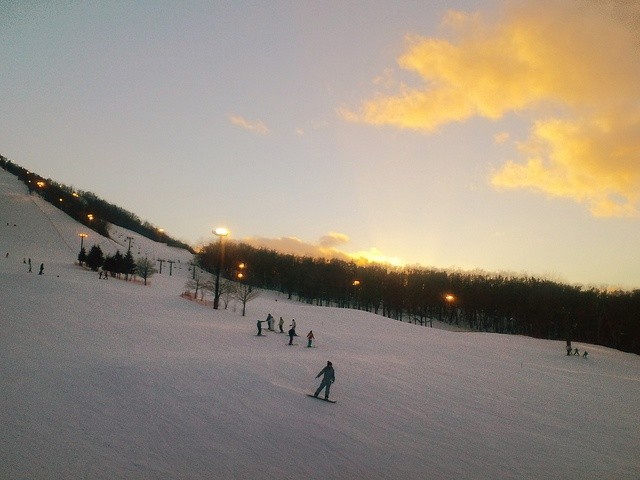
left=308, top=394, right=337, bottom=404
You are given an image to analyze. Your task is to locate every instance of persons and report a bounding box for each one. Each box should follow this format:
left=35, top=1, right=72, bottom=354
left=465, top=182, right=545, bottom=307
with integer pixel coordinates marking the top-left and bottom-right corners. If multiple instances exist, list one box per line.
left=290, top=319, right=297, bottom=335
left=266, top=313, right=275, bottom=330
left=288, top=326, right=297, bottom=345
left=582, top=350, right=589, bottom=359
left=99, top=271, right=103, bottom=279
left=278, top=317, right=284, bottom=333
left=567, top=347, right=572, bottom=356
left=105, top=272, right=108, bottom=278
left=256, top=320, right=265, bottom=336
left=306, top=330, right=315, bottom=347
left=574, top=348, right=580, bottom=356
left=314, top=361, right=336, bottom=399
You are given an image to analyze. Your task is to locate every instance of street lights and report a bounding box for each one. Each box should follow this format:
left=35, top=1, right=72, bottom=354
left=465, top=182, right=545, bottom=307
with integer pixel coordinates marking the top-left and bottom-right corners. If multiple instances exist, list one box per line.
left=238, top=274, right=244, bottom=288
left=168, top=261, right=175, bottom=276
left=157, top=260, right=166, bottom=273
left=126, top=236, right=134, bottom=251
left=447, top=295, right=455, bottom=326
left=352, top=280, right=360, bottom=310
left=238, top=263, right=244, bottom=273
left=79, top=233, right=88, bottom=264
left=213, top=227, right=228, bottom=309
left=191, top=263, right=199, bottom=279
